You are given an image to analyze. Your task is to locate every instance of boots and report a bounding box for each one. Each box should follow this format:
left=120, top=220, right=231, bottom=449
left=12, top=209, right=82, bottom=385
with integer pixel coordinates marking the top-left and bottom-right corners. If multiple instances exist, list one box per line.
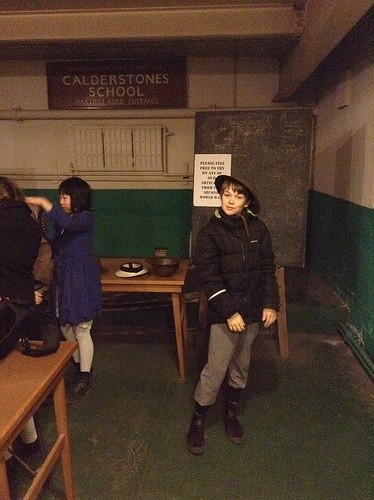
left=187, top=401, right=210, bottom=456
left=225, top=387, right=246, bottom=445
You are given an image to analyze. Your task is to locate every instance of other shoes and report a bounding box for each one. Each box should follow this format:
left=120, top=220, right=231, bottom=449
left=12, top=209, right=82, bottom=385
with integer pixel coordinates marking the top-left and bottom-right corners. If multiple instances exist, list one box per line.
left=66, top=366, right=94, bottom=404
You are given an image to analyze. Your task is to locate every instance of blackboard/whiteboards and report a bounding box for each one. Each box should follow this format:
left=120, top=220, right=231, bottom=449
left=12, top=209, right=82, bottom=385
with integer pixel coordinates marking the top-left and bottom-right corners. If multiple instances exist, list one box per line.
left=187, top=105, right=315, bottom=270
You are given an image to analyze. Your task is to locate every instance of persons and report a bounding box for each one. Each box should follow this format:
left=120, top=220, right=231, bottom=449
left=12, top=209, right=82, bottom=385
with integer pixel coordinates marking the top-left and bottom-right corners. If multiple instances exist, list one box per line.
left=24, top=177, right=102, bottom=403
left=185, top=176, right=281, bottom=453
left=0, top=176, right=54, bottom=343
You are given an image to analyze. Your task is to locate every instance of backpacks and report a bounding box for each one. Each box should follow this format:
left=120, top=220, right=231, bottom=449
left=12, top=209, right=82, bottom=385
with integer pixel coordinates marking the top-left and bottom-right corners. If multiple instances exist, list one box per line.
left=0, top=298, right=61, bottom=360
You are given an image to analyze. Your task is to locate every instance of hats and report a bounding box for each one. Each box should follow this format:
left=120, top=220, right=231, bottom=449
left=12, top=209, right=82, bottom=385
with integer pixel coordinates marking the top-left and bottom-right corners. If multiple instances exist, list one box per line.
left=214, top=173, right=261, bottom=216
left=115, top=262, right=149, bottom=278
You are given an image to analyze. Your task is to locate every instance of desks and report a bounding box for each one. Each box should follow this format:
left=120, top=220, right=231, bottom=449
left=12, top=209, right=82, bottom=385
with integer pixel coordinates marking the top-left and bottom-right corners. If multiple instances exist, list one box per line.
left=0, top=339, right=78, bottom=500
left=101, top=257, right=190, bottom=382
